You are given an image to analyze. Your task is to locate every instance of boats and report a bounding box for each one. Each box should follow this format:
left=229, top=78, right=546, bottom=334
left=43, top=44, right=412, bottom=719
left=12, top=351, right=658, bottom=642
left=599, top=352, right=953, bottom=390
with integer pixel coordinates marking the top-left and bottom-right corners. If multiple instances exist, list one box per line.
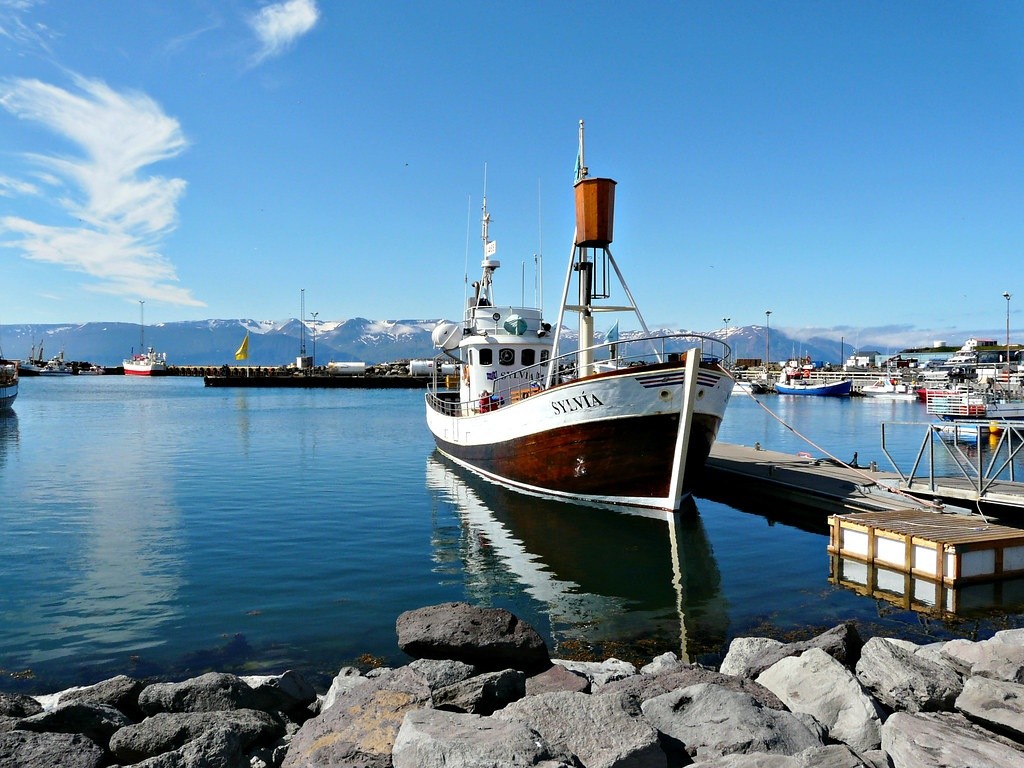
left=123, top=345, right=170, bottom=377
left=750, top=339, right=1024, bottom=434
left=0, top=337, right=106, bottom=409
left=424, top=119, right=738, bottom=514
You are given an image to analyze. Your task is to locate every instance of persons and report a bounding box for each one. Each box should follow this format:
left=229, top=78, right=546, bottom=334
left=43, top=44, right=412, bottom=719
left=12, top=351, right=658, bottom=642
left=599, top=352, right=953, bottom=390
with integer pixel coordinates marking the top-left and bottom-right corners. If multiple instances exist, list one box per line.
left=479, top=390, right=499, bottom=414
left=551, top=362, right=575, bottom=387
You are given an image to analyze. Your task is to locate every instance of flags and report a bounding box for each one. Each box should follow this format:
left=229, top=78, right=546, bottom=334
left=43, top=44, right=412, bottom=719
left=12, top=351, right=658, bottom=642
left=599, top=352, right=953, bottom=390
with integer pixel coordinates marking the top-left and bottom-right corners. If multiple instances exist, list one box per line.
left=235, top=335, right=248, bottom=360
left=604, top=320, right=618, bottom=345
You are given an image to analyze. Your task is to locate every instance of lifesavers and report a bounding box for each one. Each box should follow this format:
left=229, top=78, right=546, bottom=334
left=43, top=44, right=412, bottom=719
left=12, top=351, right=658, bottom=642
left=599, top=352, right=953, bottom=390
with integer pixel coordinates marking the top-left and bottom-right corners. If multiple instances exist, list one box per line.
left=804, top=370, right=810, bottom=377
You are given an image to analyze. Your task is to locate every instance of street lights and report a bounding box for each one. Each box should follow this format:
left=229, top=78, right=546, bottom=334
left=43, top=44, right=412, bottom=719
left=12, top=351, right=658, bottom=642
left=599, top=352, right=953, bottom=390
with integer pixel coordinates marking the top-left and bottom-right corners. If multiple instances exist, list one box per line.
left=1004, top=291, right=1012, bottom=397
left=723, top=318, right=732, bottom=362
left=765, top=311, right=772, bottom=374
left=310, top=312, right=319, bottom=367
left=139, top=301, right=146, bottom=352
